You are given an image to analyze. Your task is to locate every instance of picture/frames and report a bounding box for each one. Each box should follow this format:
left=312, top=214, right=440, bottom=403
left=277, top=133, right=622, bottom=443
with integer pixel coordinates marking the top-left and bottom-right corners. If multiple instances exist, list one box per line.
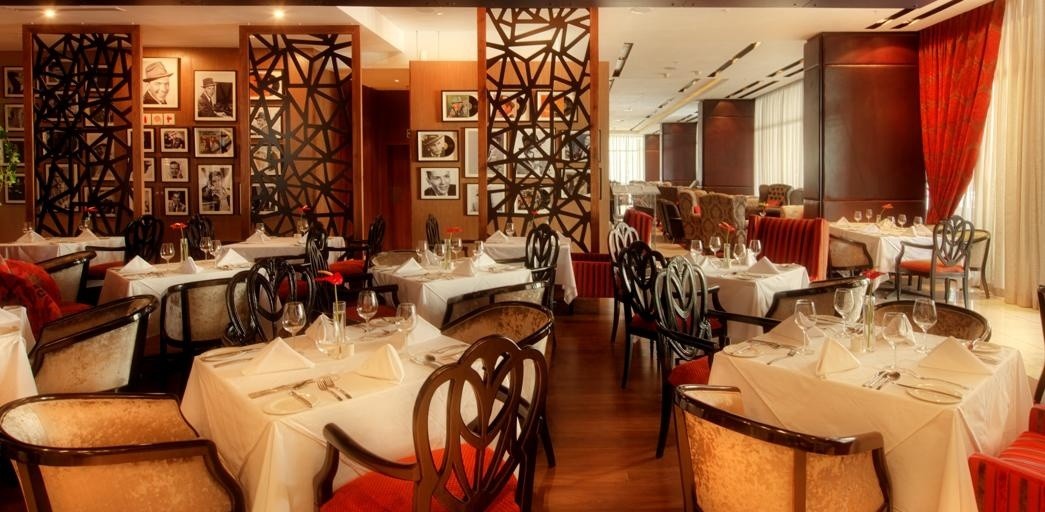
left=417, top=90, right=602, bottom=215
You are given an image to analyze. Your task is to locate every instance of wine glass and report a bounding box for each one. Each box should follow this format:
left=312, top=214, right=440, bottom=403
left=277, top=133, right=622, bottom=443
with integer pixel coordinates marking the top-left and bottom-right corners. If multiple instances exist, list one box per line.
left=709, top=236, right=721, bottom=257
left=793, top=298, right=817, bottom=355
left=733, top=243, right=747, bottom=272
left=79, top=220, right=93, bottom=231
left=314, top=316, right=342, bottom=356
left=880, top=311, right=908, bottom=371
left=416, top=236, right=485, bottom=272
left=356, top=291, right=378, bottom=335
left=282, top=302, right=307, bottom=347
left=749, top=239, right=761, bottom=259
left=912, top=297, right=938, bottom=355
left=395, top=302, right=416, bottom=350
left=21, top=222, right=32, bottom=234
left=834, top=288, right=855, bottom=337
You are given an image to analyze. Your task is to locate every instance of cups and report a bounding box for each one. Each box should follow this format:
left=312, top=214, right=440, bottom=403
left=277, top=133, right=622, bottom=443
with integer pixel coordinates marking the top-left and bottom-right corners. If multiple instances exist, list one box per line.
left=691, top=239, right=703, bottom=256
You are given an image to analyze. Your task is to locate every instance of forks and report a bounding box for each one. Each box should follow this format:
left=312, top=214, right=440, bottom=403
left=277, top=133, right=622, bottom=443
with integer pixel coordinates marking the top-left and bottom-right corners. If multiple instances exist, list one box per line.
left=317, top=380, right=343, bottom=401
left=765, top=348, right=800, bottom=366
left=324, top=377, right=351, bottom=398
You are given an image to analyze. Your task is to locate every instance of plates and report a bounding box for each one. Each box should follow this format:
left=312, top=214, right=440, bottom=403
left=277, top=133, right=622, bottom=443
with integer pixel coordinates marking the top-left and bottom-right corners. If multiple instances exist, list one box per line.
left=724, top=341, right=772, bottom=358
left=263, top=393, right=320, bottom=415
left=906, top=383, right=963, bottom=404
left=962, top=340, right=1001, bottom=353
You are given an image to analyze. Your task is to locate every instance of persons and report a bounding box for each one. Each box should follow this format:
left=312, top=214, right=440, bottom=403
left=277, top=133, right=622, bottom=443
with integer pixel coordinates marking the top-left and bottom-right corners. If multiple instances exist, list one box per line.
left=448, top=96, right=464, bottom=117
left=254, top=112, right=270, bottom=136
left=423, top=134, right=446, bottom=157
left=50, top=166, right=70, bottom=209
left=169, top=161, right=181, bottom=178
left=141, top=61, right=175, bottom=105
left=424, top=171, right=456, bottom=196
left=496, top=95, right=515, bottom=118
left=197, top=78, right=221, bottom=117
left=202, top=171, right=230, bottom=212
left=164, top=131, right=184, bottom=149
left=168, top=193, right=187, bottom=213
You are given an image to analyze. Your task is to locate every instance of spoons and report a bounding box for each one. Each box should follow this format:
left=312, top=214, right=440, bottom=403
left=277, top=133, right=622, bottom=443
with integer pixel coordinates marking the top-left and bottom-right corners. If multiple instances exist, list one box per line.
left=426, top=355, right=444, bottom=365
left=876, top=372, right=900, bottom=390
